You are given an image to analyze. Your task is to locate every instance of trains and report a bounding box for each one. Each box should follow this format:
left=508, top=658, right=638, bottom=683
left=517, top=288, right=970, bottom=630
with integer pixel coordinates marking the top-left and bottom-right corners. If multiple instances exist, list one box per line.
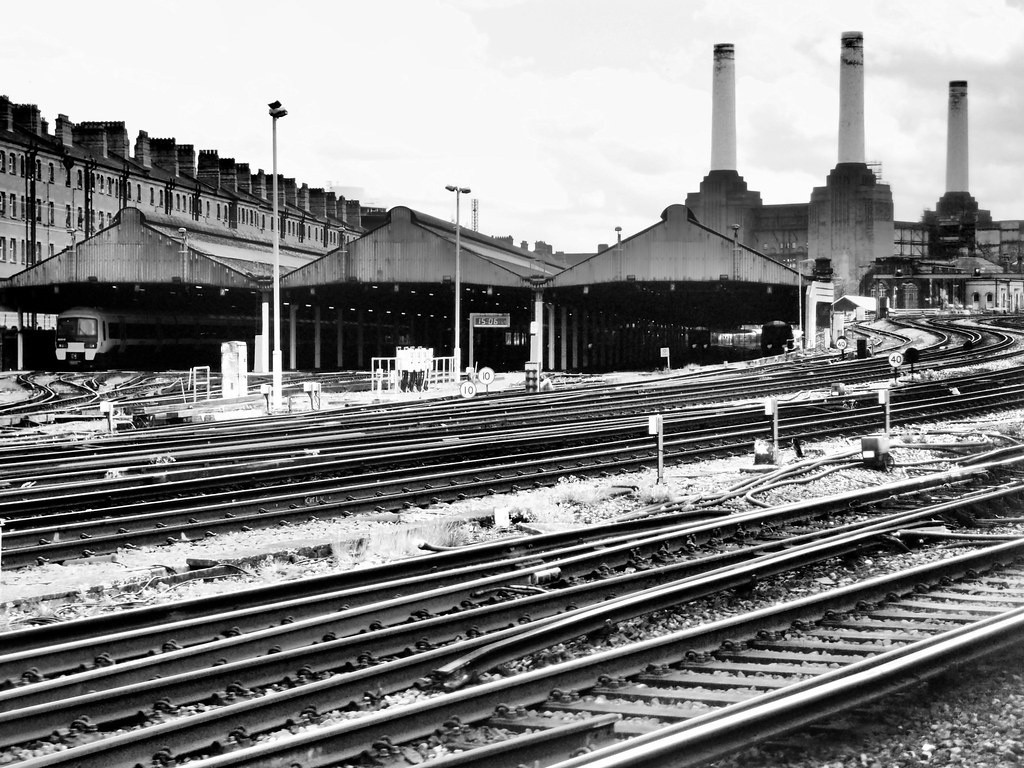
left=52, top=306, right=455, bottom=369
left=686, top=329, right=713, bottom=355
left=720, top=320, right=793, bottom=353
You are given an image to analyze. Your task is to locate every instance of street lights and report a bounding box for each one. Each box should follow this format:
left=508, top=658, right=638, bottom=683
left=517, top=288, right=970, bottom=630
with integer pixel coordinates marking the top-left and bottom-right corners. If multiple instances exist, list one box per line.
left=798, top=258, right=816, bottom=354
left=446, top=186, right=471, bottom=380
left=268, top=100, right=291, bottom=413
